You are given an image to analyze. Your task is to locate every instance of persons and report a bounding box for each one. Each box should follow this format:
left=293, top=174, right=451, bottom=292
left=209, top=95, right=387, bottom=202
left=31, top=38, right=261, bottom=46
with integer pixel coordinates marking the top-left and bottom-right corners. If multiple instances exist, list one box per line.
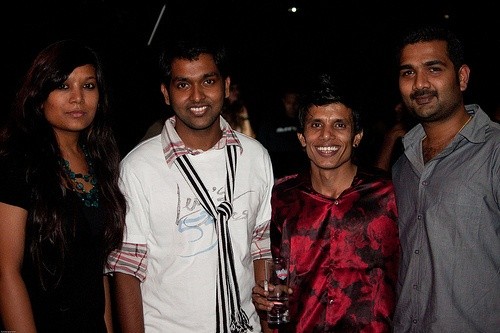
left=259, top=89, right=310, bottom=178
left=0, top=40, right=128, bottom=333
left=256, top=74, right=403, bottom=333
left=112, top=41, right=275, bottom=333
left=373, top=94, right=414, bottom=178
left=389, top=25, right=500, bottom=333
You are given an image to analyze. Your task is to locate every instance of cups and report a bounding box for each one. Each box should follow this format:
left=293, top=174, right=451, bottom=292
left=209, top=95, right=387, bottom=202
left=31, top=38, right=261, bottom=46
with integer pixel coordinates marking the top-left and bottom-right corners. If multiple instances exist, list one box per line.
left=263, top=258, right=290, bottom=328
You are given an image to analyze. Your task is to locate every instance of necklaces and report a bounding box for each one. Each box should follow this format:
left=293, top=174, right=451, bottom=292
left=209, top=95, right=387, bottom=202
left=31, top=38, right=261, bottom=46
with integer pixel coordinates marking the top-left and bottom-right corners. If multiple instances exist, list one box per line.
left=57, top=140, right=101, bottom=207
